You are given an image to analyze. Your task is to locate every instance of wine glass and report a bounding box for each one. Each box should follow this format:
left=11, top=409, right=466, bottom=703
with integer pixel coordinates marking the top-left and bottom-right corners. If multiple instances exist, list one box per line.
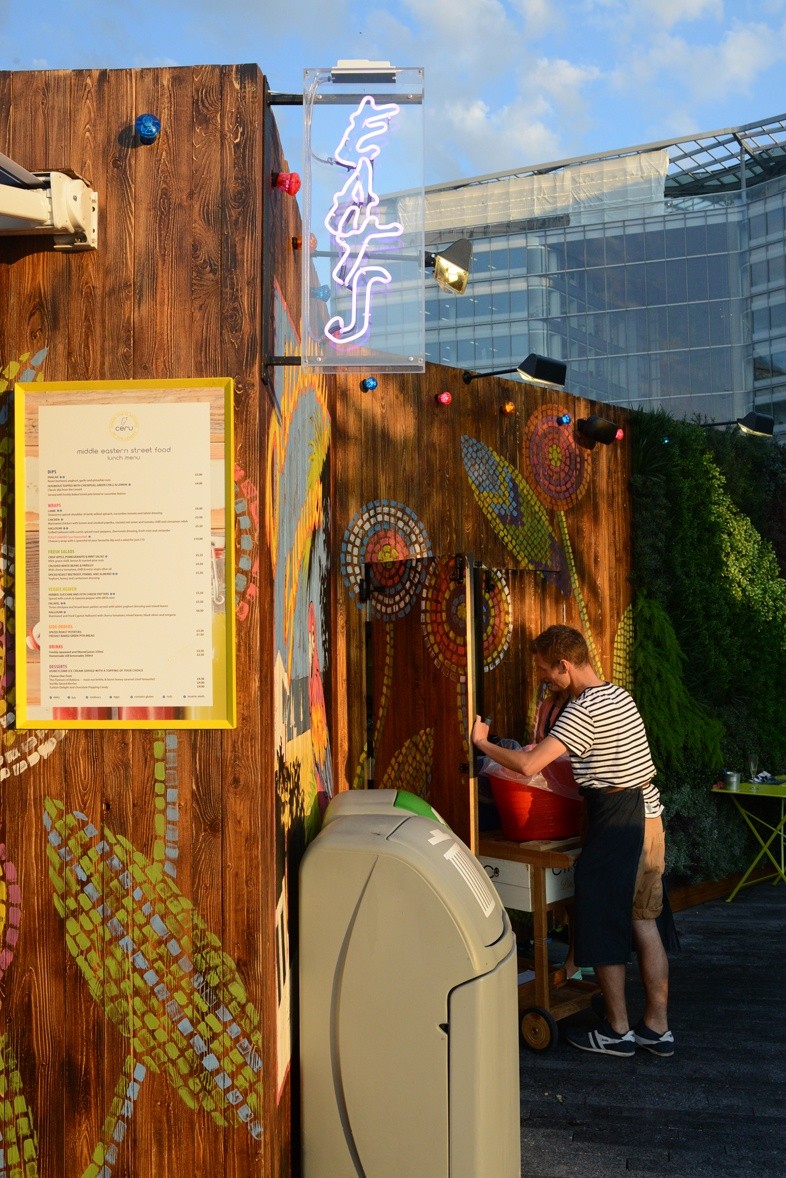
left=751, top=760, right=758, bottom=784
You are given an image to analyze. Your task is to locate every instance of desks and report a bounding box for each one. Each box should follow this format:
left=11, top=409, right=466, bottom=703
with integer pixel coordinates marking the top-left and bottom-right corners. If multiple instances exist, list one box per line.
left=711, top=774, right=786, bottom=903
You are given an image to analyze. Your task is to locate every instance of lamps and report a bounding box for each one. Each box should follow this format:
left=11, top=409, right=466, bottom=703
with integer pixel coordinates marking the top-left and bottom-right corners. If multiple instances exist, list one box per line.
left=463, top=353, right=566, bottom=390
left=699, top=411, right=774, bottom=437
left=314, top=238, right=473, bottom=294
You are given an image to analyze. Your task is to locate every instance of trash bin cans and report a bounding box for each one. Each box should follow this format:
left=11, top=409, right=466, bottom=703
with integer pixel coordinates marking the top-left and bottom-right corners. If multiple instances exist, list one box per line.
left=320, top=788, right=453, bottom=832
left=300, top=813, right=522, bottom=1178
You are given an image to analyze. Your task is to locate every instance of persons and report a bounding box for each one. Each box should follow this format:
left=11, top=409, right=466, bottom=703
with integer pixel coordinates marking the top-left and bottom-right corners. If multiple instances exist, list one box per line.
left=470, top=624, right=682, bottom=1056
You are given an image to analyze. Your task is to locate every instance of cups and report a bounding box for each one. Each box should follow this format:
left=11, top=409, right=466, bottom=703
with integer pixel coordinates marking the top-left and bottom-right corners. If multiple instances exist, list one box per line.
left=727, top=771, right=737, bottom=791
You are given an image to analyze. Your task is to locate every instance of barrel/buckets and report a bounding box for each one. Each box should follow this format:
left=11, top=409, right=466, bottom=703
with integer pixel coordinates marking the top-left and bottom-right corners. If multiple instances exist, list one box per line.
left=479, top=755, right=584, bottom=840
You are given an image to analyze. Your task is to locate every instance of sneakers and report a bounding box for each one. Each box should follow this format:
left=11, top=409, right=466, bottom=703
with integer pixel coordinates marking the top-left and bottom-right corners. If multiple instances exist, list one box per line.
left=633, top=1019, right=675, bottom=1055
left=566, top=1019, right=634, bottom=1056
